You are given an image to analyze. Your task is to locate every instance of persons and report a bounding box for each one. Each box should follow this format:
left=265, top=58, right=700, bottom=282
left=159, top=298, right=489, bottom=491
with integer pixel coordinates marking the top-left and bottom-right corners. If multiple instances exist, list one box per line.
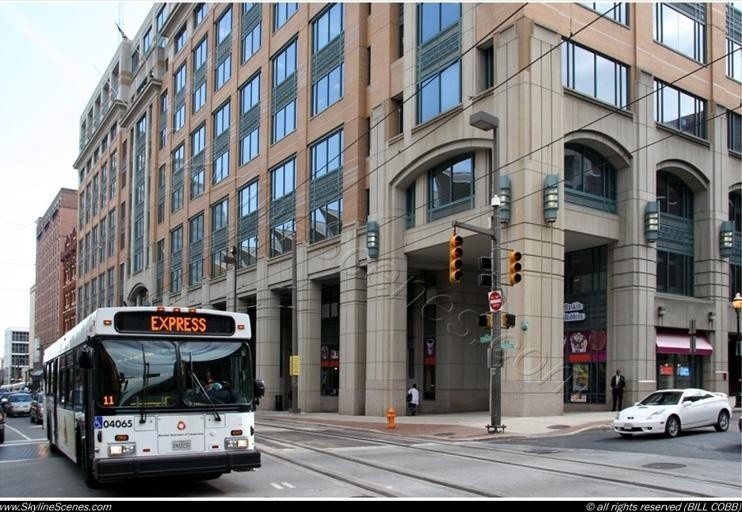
left=610, top=368, right=626, bottom=410
left=406, top=383, right=420, bottom=415
left=197, top=366, right=225, bottom=393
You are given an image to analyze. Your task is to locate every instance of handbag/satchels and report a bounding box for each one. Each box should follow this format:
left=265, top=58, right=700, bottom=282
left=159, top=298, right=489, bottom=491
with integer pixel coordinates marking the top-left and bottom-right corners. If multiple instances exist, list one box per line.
left=406, top=394, right=412, bottom=402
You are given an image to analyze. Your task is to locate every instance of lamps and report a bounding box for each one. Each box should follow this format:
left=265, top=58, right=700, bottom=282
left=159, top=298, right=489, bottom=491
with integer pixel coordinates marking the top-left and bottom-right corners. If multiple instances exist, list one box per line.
left=719, top=221, right=734, bottom=258
left=366, top=221, right=379, bottom=258
left=659, top=304, right=668, bottom=316
left=544, top=175, right=559, bottom=223
left=498, top=176, right=511, bottom=225
left=709, top=311, right=717, bottom=321
left=644, top=201, right=659, bottom=243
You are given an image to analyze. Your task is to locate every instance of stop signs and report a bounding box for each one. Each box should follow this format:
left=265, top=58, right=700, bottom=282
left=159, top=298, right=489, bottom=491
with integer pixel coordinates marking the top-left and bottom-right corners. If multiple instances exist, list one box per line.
left=487, top=290, right=504, bottom=312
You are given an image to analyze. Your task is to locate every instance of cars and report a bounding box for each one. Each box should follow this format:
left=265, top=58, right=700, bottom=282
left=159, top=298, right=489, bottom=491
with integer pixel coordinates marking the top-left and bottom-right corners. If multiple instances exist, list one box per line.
left=0, top=385, right=43, bottom=444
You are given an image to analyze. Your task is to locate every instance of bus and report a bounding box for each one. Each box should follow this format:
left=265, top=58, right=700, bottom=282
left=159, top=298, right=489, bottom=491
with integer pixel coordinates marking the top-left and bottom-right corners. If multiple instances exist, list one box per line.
left=42, top=306, right=265, bottom=491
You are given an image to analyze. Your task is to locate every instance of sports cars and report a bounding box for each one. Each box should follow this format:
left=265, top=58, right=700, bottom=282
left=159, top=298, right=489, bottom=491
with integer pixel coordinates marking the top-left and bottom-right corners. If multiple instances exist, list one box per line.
left=612, top=387, right=732, bottom=439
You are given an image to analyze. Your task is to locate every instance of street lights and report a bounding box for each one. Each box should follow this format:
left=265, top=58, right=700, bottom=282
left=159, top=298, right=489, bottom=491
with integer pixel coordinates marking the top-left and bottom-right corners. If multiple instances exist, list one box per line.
left=731, top=292, right=742, bottom=408
left=469, top=111, right=508, bottom=435
left=219, top=254, right=238, bottom=314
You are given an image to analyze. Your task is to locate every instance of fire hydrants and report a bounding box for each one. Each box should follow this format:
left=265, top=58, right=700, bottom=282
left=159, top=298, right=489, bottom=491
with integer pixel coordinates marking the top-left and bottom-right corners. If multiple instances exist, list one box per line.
left=386, top=407, right=397, bottom=431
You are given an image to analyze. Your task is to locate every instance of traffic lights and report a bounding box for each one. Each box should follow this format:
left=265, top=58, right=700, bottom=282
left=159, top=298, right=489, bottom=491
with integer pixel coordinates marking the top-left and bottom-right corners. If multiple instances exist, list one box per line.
left=477, top=273, right=492, bottom=287
left=449, top=235, right=464, bottom=284
left=477, top=255, right=492, bottom=272
left=508, top=251, right=521, bottom=286
left=477, top=314, right=492, bottom=328
left=502, top=313, right=516, bottom=327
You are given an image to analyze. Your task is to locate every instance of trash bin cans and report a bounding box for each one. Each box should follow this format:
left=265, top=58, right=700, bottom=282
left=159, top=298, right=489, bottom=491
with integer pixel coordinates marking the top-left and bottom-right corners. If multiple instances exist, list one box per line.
left=275, top=395, right=282, bottom=411
